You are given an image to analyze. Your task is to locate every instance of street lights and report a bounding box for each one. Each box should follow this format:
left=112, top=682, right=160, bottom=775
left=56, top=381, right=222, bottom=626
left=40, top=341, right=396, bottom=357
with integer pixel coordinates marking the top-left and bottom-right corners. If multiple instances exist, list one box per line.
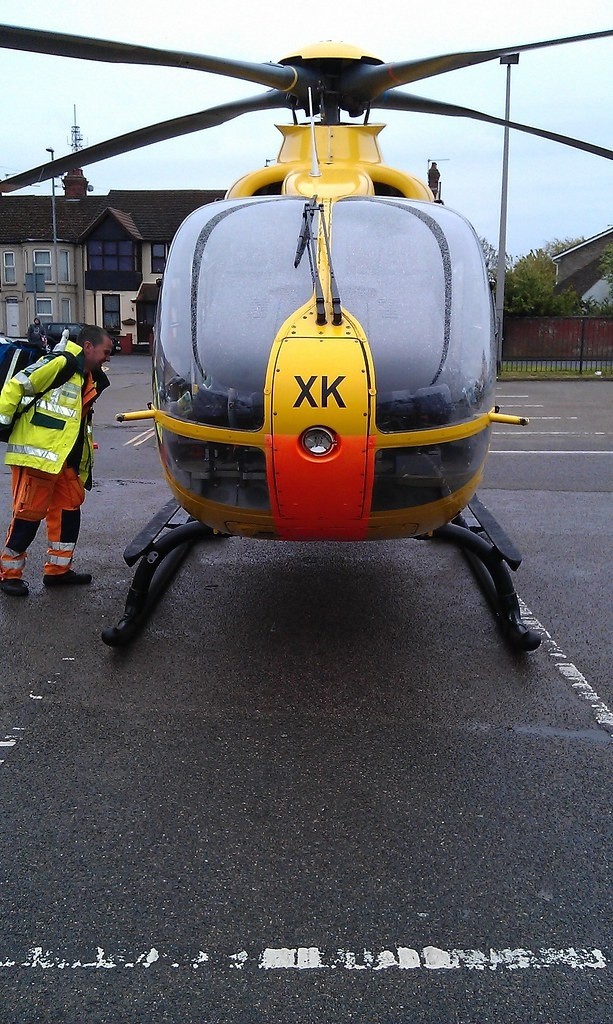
left=495, top=53, right=520, bottom=377
left=46, top=147, right=61, bottom=324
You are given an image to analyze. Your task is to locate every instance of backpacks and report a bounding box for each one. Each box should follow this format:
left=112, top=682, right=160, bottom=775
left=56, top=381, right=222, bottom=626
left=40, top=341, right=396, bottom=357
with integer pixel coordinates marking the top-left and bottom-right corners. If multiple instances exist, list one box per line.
left=0, top=340, right=79, bottom=443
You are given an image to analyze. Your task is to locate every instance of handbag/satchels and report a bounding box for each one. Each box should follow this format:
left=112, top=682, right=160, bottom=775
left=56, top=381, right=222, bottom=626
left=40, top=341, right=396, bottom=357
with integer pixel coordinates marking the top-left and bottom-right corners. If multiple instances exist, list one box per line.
left=41, top=335, right=47, bottom=347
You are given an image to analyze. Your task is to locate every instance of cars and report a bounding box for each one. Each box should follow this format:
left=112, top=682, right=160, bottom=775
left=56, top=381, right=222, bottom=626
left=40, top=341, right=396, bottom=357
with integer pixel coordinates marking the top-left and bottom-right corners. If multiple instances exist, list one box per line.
left=41, top=323, right=121, bottom=355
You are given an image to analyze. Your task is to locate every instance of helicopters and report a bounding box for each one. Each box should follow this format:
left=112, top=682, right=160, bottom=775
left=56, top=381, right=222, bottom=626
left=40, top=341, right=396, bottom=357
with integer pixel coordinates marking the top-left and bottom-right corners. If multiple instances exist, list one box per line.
left=0, top=25, right=613, bottom=654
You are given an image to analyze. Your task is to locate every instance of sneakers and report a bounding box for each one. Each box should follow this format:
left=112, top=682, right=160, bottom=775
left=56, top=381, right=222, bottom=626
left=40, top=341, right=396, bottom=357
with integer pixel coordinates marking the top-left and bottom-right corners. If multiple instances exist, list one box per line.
left=0, top=577, right=29, bottom=597
left=42, top=568, right=92, bottom=587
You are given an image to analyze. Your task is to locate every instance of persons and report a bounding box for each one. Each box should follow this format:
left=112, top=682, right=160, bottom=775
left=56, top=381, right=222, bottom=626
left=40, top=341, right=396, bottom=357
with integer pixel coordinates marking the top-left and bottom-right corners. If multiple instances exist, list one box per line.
left=426, top=162, right=441, bottom=200
left=27, top=318, right=46, bottom=344
left=0, top=325, right=113, bottom=596
